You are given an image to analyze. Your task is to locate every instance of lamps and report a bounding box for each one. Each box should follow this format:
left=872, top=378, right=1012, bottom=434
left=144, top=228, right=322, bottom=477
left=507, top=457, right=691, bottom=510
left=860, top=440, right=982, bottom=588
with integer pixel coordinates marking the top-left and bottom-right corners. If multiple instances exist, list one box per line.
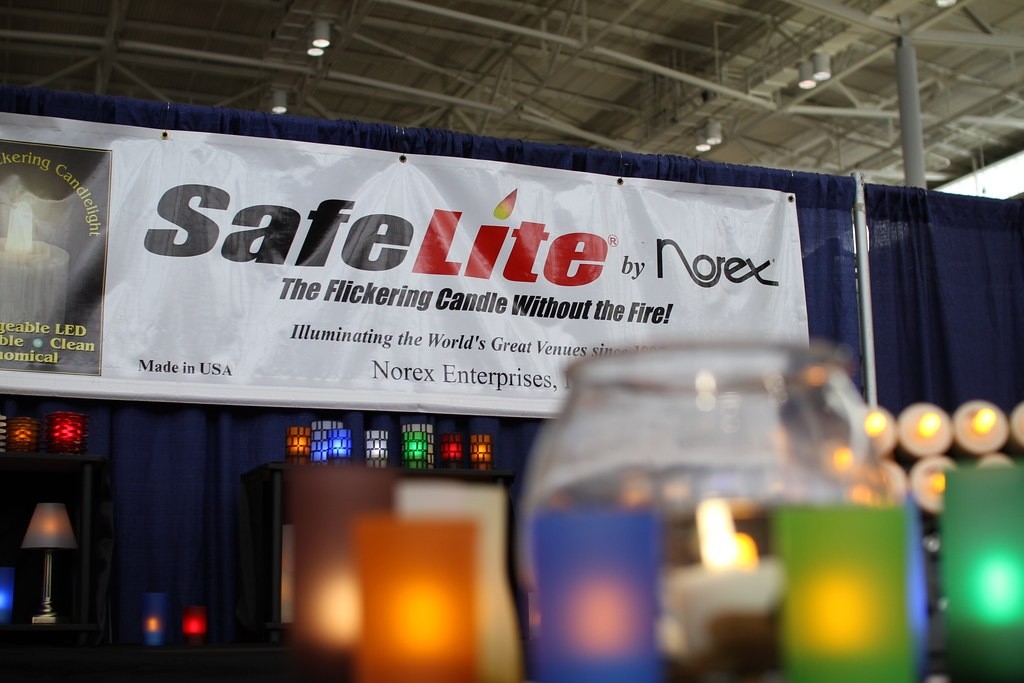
left=307, top=23, right=330, bottom=58
left=8, top=416, right=38, bottom=451
left=694, top=121, right=722, bottom=152
left=286, top=421, right=492, bottom=473
left=796, top=52, right=831, bottom=90
left=268, top=90, right=287, bottom=114
left=23, top=504, right=76, bottom=623
left=44, top=412, right=86, bottom=454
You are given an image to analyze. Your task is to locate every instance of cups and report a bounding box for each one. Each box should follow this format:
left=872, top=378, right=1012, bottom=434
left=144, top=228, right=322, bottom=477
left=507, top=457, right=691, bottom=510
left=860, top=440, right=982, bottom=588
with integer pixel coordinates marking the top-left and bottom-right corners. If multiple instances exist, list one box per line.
left=510, top=340, right=888, bottom=681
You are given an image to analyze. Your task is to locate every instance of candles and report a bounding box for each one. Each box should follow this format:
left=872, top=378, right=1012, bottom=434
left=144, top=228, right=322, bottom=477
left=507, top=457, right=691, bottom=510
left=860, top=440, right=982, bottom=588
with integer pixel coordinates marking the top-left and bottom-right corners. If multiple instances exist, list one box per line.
left=772, top=507, right=925, bottom=682
left=350, top=518, right=478, bottom=682
left=944, top=469, right=1024, bottom=683
left=533, top=509, right=664, bottom=682
left=180, top=596, right=206, bottom=644
left=684, top=500, right=780, bottom=683
left=294, top=466, right=392, bottom=661
left=136, top=592, right=167, bottom=644
left=2, top=198, right=69, bottom=356
left=0, top=568, right=14, bottom=622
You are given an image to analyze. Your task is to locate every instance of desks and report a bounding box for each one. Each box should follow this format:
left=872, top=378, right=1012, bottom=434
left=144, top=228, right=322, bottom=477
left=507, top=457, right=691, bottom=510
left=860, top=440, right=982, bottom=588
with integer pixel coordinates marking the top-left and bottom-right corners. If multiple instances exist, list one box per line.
left=0, top=622, right=96, bottom=641
left=0, top=645, right=299, bottom=682
